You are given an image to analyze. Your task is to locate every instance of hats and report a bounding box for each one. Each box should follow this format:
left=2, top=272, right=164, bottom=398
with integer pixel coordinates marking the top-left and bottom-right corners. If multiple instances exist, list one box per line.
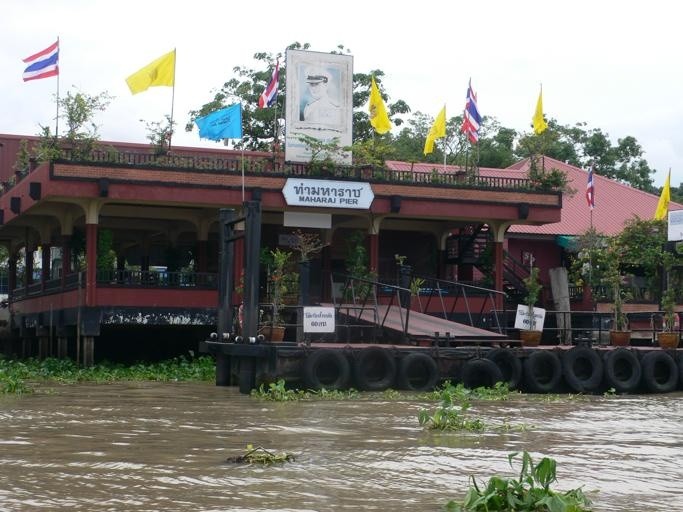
left=305, top=66, right=331, bottom=85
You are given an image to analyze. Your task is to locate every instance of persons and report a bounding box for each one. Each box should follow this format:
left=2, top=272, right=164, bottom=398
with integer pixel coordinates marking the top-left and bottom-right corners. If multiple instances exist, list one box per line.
left=300, top=66, right=341, bottom=126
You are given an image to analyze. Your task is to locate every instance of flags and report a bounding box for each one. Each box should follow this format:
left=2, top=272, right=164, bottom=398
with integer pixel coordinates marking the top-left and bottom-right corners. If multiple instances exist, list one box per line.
left=123, top=47, right=175, bottom=94
left=529, top=91, right=548, bottom=135
left=191, top=101, right=243, bottom=145
left=458, top=85, right=484, bottom=144
left=422, top=105, right=447, bottom=156
left=647, top=171, right=671, bottom=223
left=19, top=37, right=58, bottom=83
left=255, top=59, right=279, bottom=110
left=583, top=165, right=597, bottom=213
left=364, top=75, right=392, bottom=136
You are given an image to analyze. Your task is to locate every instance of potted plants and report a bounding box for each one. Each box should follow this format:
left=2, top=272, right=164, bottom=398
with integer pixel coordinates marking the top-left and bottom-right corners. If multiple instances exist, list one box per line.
left=518, top=267, right=544, bottom=348
left=605, top=249, right=681, bottom=348
left=258, top=247, right=292, bottom=341
left=453, top=170, right=465, bottom=184
